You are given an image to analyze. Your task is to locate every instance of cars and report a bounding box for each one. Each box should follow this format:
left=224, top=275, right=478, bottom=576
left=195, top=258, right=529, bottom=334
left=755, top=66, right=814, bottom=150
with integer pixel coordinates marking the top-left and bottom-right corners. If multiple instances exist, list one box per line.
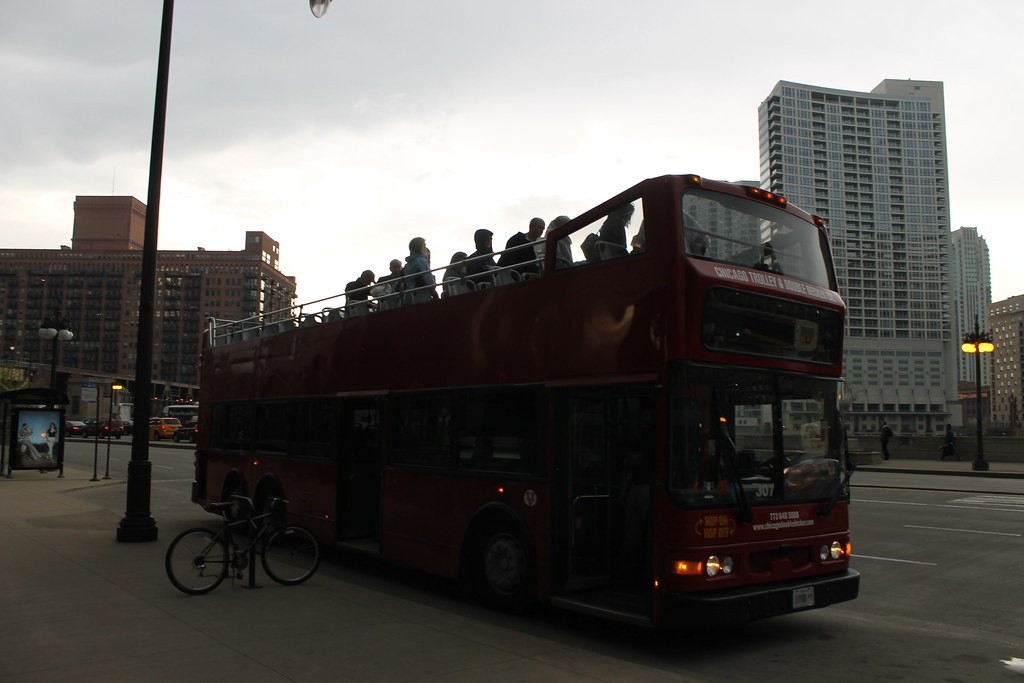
left=123, top=421, right=134, bottom=435
left=64, top=420, right=85, bottom=436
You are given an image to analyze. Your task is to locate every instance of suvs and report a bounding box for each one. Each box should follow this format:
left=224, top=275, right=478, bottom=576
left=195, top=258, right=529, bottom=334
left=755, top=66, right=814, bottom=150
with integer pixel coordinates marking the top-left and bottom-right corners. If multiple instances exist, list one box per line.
left=174, top=422, right=198, bottom=442
left=148, top=417, right=182, bottom=440
left=82, top=418, right=124, bottom=439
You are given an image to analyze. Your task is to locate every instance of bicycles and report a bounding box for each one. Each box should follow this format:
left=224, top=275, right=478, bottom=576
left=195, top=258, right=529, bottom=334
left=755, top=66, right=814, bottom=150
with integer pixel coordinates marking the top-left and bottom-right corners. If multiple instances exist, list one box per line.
left=164, top=494, right=318, bottom=594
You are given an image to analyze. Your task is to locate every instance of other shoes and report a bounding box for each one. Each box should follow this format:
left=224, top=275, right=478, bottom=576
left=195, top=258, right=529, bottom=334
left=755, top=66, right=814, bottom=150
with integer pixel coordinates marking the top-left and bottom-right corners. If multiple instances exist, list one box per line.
left=883, top=456, right=889, bottom=460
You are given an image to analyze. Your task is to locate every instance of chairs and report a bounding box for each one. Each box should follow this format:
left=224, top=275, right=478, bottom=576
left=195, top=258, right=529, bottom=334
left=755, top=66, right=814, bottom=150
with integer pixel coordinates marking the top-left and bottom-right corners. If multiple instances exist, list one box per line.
left=224, top=241, right=632, bottom=345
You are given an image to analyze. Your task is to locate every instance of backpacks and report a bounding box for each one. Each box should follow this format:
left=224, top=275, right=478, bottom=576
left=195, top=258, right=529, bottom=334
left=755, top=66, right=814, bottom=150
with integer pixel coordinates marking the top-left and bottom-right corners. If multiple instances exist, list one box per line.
left=885, top=427, right=893, bottom=438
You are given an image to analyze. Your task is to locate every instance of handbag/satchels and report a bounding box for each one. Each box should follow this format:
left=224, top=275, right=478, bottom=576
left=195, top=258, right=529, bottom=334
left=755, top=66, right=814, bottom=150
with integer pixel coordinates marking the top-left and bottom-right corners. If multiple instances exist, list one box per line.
left=581, top=233, right=601, bottom=264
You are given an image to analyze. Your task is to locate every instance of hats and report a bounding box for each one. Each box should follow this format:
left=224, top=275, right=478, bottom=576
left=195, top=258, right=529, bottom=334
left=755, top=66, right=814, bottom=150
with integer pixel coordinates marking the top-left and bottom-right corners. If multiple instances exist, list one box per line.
left=362, top=270, right=377, bottom=284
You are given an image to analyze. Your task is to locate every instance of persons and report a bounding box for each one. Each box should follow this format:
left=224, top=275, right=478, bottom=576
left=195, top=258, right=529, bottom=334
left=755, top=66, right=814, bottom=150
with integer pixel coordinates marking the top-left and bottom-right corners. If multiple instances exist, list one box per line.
left=937, top=424, right=961, bottom=461
left=879, top=421, right=893, bottom=460
left=544, top=216, right=573, bottom=268
left=442, top=253, right=467, bottom=294
left=631, top=210, right=711, bottom=254
left=345, top=238, right=440, bottom=310
left=465, top=229, right=495, bottom=285
left=46, top=423, right=57, bottom=462
left=20, top=423, right=43, bottom=462
left=496, top=218, right=545, bottom=274
left=591, top=203, right=634, bottom=260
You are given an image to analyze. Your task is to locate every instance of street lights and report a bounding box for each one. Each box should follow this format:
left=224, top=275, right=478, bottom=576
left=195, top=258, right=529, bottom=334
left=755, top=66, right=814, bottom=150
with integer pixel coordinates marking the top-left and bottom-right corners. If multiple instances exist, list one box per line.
left=103, top=382, right=123, bottom=479
left=962, top=333, right=994, bottom=470
left=37, top=311, right=74, bottom=390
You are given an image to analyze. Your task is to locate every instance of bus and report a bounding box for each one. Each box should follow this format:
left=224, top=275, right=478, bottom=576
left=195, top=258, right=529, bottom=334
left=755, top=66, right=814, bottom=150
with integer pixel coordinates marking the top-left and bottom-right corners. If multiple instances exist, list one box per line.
left=189, top=174, right=862, bottom=635
left=162, top=404, right=199, bottom=425
